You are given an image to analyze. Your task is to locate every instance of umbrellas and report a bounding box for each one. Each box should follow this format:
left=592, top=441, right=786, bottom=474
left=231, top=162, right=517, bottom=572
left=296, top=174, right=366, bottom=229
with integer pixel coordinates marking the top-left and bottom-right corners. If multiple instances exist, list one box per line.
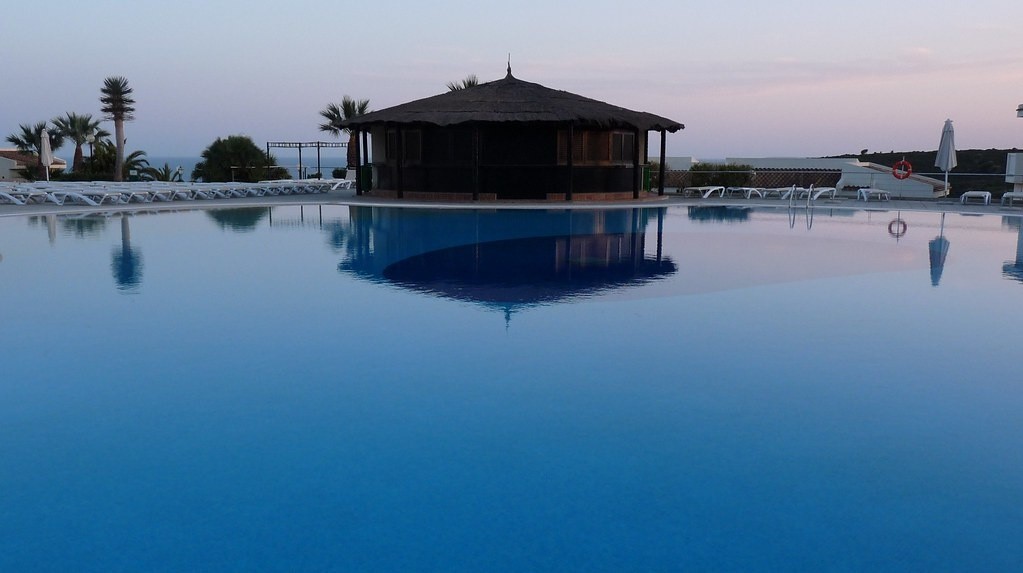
left=40, top=129, right=53, bottom=182
left=935, top=118, right=958, bottom=200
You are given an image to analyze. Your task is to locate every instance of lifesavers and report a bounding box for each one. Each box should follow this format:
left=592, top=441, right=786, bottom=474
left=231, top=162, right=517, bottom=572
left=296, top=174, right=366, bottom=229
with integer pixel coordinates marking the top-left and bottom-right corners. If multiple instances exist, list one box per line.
left=892, top=160, right=913, bottom=180
left=887, top=218, right=908, bottom=237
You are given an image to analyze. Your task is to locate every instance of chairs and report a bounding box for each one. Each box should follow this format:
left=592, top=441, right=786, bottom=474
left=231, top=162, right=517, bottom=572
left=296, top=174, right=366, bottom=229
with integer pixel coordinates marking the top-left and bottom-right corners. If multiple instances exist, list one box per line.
left=857, top=189, right=891, bottom=203
left=1001, top=192, right=1023, bottom=207
left=960, top=191, right=991, bottom=205
left=0, top=178, right=352, bottom=206
left=345, top=170, right=356, bottom=188
left=684, top=186, right=837, bottom=200
